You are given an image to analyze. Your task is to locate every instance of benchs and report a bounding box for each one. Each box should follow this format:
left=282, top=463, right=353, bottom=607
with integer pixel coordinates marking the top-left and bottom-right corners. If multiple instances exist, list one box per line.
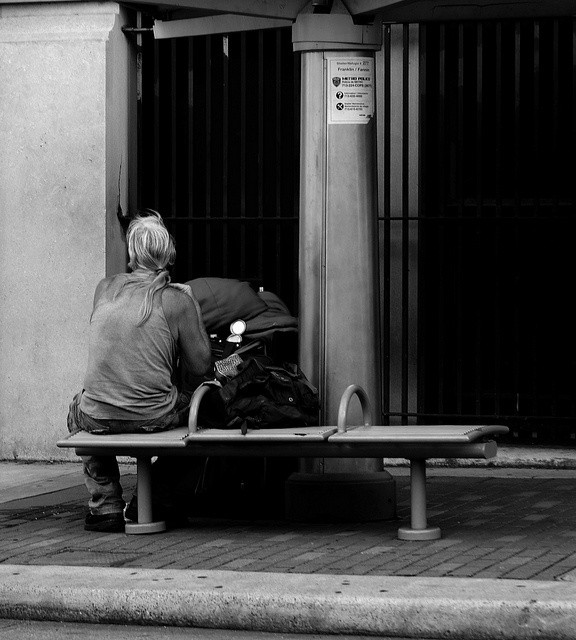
left=54, top=380, right=512, bottom=539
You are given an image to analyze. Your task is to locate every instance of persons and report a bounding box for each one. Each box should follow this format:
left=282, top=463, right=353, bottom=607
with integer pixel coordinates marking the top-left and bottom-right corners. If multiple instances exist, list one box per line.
left=67, top=209, right=211, bottom=533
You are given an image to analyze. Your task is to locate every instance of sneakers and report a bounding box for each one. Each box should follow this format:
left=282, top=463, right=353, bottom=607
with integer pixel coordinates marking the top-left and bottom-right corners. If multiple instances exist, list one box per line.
left=84, top=512, right=126, bottom=532
left=125, top=497, right=138, bottom=521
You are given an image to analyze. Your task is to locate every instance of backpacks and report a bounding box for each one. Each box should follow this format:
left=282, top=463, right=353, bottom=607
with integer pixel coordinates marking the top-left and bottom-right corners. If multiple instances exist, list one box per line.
left=215, top=354, right=320, bottom=434
